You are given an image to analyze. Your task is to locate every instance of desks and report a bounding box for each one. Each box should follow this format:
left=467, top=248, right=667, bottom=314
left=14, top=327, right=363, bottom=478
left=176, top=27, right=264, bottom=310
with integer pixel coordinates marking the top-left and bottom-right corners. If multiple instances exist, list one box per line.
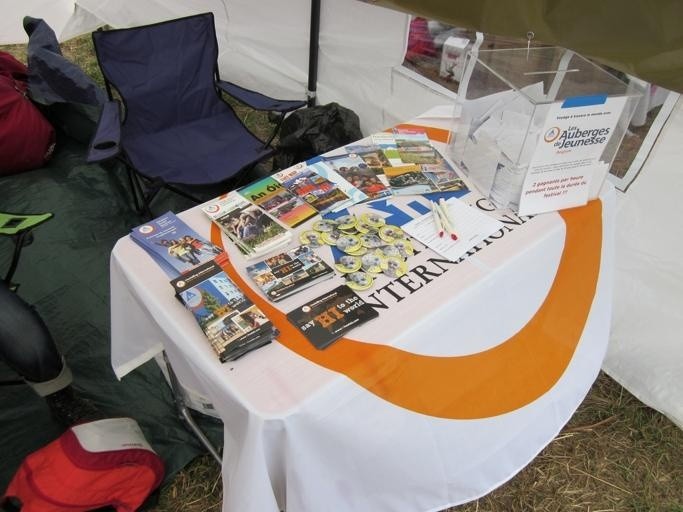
left=108, top=103, right=617, bottom=512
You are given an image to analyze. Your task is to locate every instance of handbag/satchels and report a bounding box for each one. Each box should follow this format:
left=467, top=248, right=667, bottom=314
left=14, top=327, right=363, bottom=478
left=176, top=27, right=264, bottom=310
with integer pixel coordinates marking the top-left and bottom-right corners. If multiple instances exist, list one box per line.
left=4, top=417, right=166, bottom=512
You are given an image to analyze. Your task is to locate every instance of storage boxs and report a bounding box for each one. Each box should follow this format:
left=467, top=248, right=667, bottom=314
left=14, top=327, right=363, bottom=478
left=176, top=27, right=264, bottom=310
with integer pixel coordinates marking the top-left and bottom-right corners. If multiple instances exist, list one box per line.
left=445, top=46, right=643, bottom=210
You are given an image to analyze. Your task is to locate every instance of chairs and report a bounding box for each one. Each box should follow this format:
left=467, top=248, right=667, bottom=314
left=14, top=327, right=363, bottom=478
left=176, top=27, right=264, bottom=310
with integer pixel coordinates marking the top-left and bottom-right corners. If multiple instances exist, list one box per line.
left=0, top=213, right=75, bottom=436
left=85, top=12, right=309, bottom=225
left=9, top=15, right=112, bottom=147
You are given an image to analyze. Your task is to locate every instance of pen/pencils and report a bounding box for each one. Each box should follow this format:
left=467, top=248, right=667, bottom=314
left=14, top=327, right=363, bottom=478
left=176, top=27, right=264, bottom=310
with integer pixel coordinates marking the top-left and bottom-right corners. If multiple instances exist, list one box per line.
left=429, top=197, right=458, bottom=240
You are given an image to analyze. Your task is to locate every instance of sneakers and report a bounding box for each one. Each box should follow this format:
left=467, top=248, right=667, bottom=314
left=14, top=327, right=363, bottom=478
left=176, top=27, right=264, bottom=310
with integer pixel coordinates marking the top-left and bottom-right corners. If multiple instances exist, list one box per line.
left=48, top=386, right=105, bottom=425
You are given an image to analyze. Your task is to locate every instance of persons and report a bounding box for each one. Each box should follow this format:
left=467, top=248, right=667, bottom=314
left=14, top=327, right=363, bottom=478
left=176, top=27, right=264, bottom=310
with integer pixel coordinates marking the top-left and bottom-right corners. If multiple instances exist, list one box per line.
left=1, top=280, right=109, bottom=431
left=151, top=233, right=220, bottom=266
left=334, top=166, right=384, bottom=195
left=220, top=208, right=272, bottom=240
